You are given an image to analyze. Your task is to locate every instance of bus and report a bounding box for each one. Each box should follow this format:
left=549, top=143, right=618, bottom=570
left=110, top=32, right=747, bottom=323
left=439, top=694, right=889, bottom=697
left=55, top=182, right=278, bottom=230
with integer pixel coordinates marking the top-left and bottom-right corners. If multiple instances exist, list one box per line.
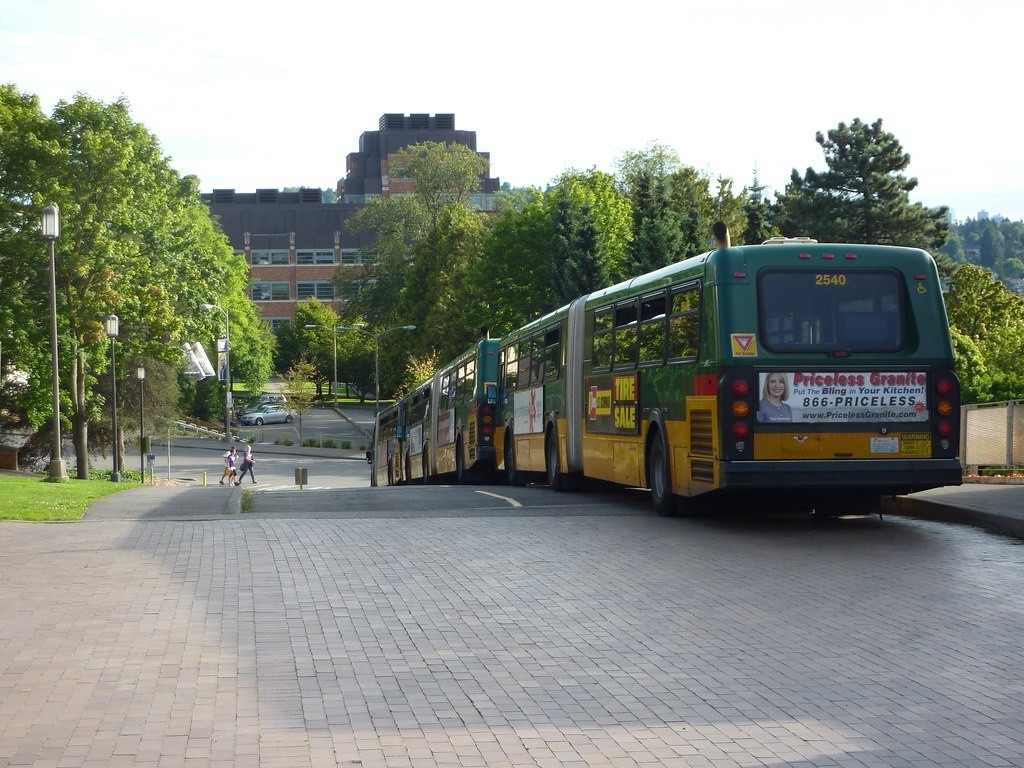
left=486, top=222, right=964, bottom=516
left=366, top=402, right=402, bottom=488
left=396, top=338, right=503, bottom=486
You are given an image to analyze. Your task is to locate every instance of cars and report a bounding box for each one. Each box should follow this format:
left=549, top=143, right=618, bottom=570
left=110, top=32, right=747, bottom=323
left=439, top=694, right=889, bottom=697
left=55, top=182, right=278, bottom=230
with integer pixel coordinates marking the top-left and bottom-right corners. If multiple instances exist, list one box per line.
left=235, top=394, right=298, bottom=426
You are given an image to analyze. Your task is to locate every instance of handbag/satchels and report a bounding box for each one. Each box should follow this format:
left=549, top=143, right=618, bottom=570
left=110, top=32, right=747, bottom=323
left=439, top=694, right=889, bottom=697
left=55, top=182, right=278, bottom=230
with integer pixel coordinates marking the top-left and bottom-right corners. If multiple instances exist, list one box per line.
left=240, top=463, right=245, bottom=471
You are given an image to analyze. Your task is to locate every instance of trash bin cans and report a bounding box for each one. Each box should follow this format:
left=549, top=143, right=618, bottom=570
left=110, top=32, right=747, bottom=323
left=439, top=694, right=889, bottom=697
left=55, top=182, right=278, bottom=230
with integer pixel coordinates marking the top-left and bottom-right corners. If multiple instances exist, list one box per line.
left=0, top=445, right=18, bottom=470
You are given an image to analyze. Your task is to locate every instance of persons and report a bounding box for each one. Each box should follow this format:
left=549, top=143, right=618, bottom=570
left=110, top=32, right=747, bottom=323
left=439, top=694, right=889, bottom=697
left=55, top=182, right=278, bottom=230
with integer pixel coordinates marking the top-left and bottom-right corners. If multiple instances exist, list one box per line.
left=219, top=447, right=241, bottom=485
left=758, top=373, right=793, bottom=423
left=225, top=448, right=236, bottom=487
left=238, top=445, right=257, bottom=483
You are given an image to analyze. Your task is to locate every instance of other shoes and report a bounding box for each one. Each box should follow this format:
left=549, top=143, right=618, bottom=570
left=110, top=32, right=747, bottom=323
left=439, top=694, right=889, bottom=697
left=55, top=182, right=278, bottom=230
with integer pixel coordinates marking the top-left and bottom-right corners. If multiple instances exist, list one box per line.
left=253, top=482, right=257, bottom=484
left=220, top=482, right=225, bottom=484
left=235, top=483, right=240, bottom=486
left=239, top=482, right=242, bottom=483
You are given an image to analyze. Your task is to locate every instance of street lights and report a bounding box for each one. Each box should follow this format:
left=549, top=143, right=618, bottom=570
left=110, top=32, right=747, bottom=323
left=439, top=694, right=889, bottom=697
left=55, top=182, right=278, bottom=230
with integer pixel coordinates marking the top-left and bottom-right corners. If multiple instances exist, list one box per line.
left=305, top=323, right=366, bottom=407
left=337, top=325, right=416, bottom=414
left=201, top=303, right=232, bottom=443
left=136, top=363, right=147, bottom=484
left=42, top=204, right=66, bottom=480
left=106, top=313, right=121, bottom=482
